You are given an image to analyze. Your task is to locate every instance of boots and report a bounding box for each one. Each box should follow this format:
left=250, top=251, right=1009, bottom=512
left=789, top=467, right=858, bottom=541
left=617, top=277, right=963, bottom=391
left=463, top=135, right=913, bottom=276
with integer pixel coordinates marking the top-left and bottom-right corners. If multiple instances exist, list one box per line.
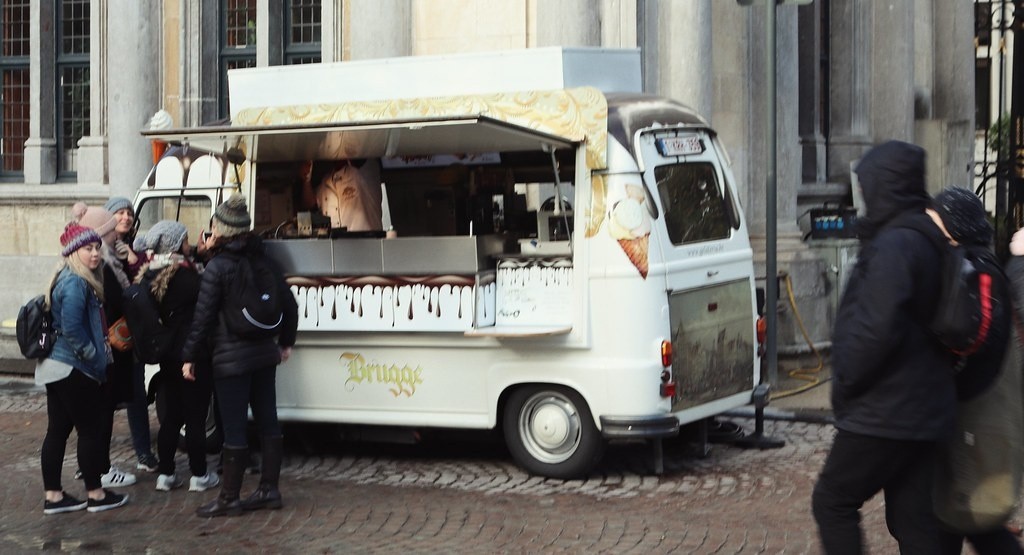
left=240, top=437, right=284, bottom=511
left=197, top=445, right=248, bottom=513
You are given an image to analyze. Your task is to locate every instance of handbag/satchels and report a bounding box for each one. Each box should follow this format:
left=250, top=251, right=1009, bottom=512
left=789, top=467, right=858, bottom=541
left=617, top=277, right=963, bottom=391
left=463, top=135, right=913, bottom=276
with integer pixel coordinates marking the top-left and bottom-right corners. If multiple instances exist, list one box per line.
left=929, top=321, right=1024, bottom=533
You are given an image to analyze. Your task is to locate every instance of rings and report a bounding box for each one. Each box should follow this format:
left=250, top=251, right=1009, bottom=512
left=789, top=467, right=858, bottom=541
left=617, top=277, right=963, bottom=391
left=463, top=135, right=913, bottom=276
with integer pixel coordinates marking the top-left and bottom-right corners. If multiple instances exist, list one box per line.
left=183, top=373, right=186, bottom=375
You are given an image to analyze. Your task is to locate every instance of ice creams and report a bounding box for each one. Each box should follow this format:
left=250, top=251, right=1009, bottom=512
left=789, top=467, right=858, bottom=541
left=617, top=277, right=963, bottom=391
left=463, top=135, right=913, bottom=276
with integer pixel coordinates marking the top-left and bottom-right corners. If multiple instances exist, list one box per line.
left=149, top=109, right=174, bottom=166
left=608, top=184, right=651, bottom=281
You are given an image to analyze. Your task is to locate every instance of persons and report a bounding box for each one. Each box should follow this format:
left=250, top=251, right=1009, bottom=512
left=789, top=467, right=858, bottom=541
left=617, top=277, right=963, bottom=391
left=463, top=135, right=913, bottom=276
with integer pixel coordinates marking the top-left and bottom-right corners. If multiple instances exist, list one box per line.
left=73, top=202, right=135, bottom=488
left=210, top=214, right=260, bottom=477
left=133, top=221, right=220, bottom=492
left=34, top=221, right=128, bottom=516
left=811, top=139, right=1024, bottom=555
left=181, top=193, right=298, bottom=519
left=74, top=197, right=159, bottom=480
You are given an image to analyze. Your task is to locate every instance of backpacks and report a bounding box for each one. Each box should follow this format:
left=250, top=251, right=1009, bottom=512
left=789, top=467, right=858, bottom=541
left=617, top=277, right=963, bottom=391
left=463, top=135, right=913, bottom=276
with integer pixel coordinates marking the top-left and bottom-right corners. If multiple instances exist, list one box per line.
left=218, top=252, right=284, bottom=340
left=123, top=279, right=190, bottom=363
left=15, top=265, right=89, bottom=360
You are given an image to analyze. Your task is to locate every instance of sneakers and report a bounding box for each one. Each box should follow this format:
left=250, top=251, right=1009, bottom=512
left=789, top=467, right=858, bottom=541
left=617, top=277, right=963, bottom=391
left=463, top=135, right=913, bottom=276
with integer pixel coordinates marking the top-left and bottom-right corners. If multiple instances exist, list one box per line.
left=191, top=470, right=219, bottom=492
left=99, top=467, right=138, bottom=487
left=155, top=470, right=184, bottom=491
left=86, top=487, right=132, bottom=513
left=43, top=492, right=89, bottom=514
left=136, top=456, right=160, bottom=471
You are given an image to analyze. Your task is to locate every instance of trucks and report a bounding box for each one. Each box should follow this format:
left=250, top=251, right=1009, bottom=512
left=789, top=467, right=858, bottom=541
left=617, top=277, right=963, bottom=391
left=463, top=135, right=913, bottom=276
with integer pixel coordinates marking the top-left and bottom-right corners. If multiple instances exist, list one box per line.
left=125, top=88, right=771, bottom=480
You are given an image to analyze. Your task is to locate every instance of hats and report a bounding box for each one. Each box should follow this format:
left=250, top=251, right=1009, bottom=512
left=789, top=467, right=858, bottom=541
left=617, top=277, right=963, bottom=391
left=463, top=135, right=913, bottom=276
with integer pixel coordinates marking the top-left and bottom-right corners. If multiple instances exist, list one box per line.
left=214, top=190, right=251, bottom=238
left=104, top=197, right=135, bottom=216
left=922, top=184, right=995, bottom=252
left=132, top=221, right=189, bottom=256
left=61, top=222, right=104, bottom=256
left=72, top=202, right=118, bottom=236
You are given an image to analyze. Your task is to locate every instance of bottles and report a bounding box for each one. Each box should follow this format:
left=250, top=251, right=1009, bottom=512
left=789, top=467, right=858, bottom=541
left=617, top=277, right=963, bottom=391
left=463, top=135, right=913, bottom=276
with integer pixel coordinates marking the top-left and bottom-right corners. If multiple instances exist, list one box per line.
left=385, top=225, right=397, bottom=237
